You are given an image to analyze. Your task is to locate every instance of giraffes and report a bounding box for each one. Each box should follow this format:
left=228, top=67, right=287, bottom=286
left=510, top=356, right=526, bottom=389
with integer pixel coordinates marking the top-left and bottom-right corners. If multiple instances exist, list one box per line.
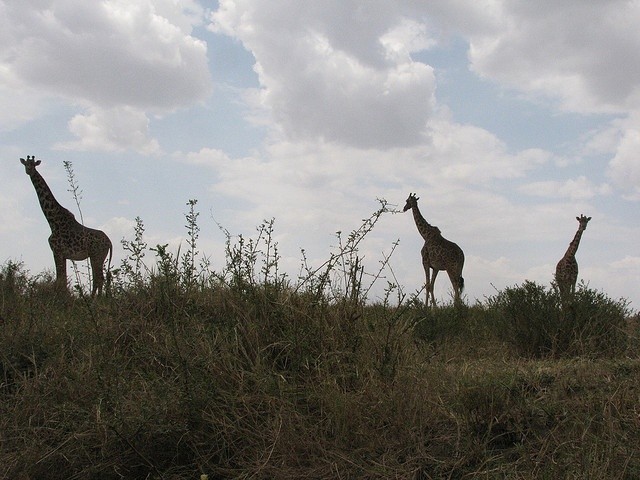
left=402, top=193, right=464, bottom=308
left=20, top=155, right=112, bottom=300
left=556, top=214, right=592, bottom=307
